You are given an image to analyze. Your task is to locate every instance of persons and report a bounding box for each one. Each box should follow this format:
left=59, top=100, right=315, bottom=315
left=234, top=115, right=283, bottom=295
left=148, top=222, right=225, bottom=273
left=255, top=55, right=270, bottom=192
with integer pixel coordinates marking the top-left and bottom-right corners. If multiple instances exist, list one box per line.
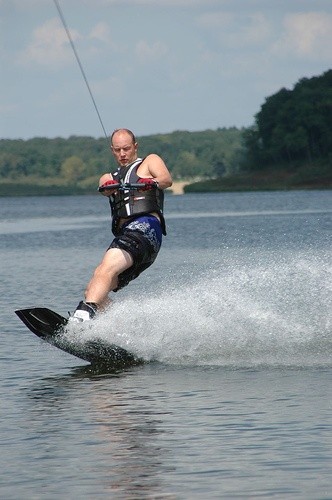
left=63, top=128, right=173, bottom=339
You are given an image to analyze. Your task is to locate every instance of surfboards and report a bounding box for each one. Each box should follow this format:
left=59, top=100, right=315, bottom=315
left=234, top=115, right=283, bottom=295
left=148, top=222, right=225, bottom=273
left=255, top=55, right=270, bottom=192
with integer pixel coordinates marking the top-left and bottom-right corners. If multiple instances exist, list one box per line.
left=15, top=307, right=134, bottom=364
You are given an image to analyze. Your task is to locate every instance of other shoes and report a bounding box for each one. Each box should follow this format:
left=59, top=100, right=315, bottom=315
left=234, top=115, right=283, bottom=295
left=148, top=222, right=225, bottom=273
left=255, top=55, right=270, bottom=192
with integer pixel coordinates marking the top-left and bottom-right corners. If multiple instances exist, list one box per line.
left=63, top=301, right=100, bottom=329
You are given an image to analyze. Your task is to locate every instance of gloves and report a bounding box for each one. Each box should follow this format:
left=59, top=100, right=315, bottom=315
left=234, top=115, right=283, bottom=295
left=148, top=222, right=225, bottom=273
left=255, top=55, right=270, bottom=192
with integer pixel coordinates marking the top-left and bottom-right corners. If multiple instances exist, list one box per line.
left=100, top=179, right=119, bottom=195
left=136, top=178, right=159, bottom=194
